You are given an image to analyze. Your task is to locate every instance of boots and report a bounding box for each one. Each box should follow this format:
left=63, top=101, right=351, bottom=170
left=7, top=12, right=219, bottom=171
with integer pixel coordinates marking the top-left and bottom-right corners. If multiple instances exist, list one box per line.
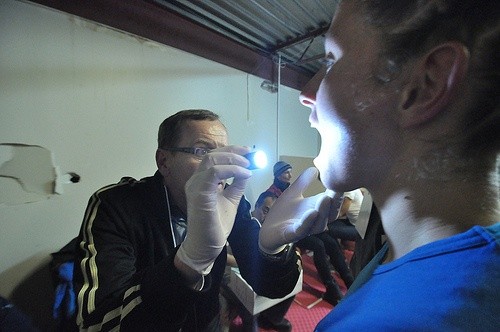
left=313, top=249, right=355, bottom=306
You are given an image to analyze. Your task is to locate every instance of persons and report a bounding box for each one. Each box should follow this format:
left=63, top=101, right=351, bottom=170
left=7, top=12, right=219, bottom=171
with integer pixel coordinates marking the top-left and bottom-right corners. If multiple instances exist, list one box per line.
left=251, top=191, right=295, bottom=332
left=327, top=189, right=364, bottom=240
left=299, top=1, right=500, bottom=332
left=268, top=160, right=356, bottom=308
left=73, top=110, right=345, bottom=332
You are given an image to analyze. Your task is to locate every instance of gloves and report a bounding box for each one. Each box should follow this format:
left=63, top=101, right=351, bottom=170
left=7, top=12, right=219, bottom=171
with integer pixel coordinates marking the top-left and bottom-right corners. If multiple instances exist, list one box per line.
left=175, top=144, right=251, bottom=277
left=258, top=167, right=344, bottom=255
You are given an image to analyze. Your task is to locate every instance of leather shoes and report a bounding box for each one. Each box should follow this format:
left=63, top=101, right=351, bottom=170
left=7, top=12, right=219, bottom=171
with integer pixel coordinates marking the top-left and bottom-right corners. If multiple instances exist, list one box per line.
left=270, top=318, right=292, bottom=332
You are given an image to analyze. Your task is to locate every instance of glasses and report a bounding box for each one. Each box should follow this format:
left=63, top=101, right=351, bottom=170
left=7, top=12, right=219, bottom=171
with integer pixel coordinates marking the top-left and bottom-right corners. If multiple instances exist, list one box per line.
left=166, top=146, right=208, bottom=158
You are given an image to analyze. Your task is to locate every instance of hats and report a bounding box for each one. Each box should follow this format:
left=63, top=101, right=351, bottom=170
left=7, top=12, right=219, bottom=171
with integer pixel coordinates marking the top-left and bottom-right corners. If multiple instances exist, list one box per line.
left=272, top=161, right=292, bottom=177
